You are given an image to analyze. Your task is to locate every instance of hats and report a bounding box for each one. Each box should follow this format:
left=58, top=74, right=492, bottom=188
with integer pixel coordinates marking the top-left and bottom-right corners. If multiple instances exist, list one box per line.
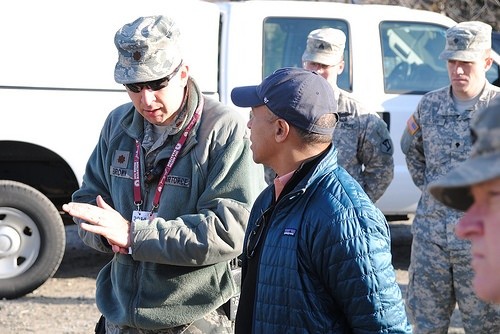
left=437, top=20, right=492, bottom=63
left=112, top=14, right=185, bottom=84
left=426, top=97, right=500, bottom=212
left=231, top=67, right=339, bottom=135
left=302, top=27, right=346, bottom=66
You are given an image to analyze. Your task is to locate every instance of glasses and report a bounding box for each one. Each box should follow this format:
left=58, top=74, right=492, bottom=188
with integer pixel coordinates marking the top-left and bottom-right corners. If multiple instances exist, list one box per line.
left=244, top=204, right=274, bottom=260
left=304, top=60, right=343, bottom=69
left=122, top=59, right=185, bottom=93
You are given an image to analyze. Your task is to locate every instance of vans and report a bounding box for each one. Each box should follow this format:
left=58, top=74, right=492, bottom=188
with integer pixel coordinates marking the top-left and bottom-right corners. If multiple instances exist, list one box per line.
left=0, top=0, right=498, bottom=298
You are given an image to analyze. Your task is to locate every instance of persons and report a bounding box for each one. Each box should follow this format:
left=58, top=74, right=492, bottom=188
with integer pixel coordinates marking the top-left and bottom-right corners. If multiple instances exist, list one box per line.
left=231, top=67, right=414, bottom=334
left=401, top=21, right=500, bottom=334
left=62, top=15, right=268, bottom=334
left=427, top=93, right=499, bottom=309
left=263, top=28, right=395, bottom=202
